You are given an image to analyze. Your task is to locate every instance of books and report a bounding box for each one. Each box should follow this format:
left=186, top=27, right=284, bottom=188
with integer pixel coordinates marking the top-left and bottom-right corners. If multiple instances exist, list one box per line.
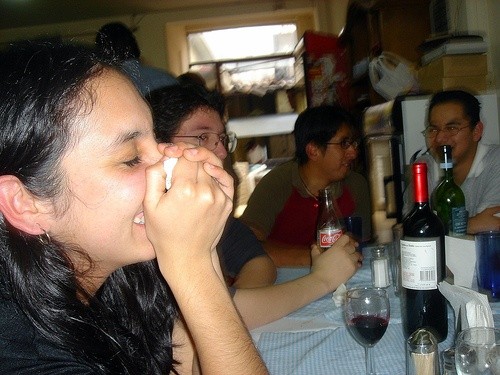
left=421, top=41, right=488, bottom=63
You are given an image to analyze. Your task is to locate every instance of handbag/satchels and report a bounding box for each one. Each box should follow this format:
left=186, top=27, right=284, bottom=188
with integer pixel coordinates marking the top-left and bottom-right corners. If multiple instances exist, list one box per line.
left=368, top=50, right=421, bottom=101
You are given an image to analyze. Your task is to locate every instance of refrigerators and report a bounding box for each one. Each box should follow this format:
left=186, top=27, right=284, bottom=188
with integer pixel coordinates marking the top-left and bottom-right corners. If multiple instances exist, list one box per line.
left=291, top=29, right=371, bottom=242
left=351, top=91, right=499, bottom=242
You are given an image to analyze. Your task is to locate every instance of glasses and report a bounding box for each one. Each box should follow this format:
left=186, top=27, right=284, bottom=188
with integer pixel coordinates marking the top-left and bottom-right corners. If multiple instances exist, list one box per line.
left=326, top=138, right=360, bottom=151
left=421, top=125, right=469, bottom=139
left=172, top=131, right=239, bottom=154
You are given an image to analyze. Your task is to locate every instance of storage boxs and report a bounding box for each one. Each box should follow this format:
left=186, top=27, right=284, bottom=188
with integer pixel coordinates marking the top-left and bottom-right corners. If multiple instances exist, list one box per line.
left=417, top=55, right=490, bottom=93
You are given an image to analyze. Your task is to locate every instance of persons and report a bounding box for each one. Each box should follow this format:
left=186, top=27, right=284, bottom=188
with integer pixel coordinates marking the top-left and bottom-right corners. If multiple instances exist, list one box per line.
left=145, top=83, right=363, bottom=329
left=402, top=90, right=500, bottom=241
left=95, top=22, right=181, bottom=94
left=238, top=105, right=372, bottom=270
left=0, top=38, right=269, bottom=375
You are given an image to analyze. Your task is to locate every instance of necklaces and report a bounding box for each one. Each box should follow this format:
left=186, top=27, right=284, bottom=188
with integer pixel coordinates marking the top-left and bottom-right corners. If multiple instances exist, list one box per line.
left=298, top=175, right=319, bottom=200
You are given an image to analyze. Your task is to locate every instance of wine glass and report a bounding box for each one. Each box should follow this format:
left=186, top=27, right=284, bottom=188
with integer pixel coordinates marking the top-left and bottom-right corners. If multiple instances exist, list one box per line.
left=345, top=288, right=392, bottom=375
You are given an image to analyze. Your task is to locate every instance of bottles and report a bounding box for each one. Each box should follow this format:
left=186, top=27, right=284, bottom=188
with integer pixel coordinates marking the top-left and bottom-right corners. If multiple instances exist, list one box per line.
left=316, top=188, right=343, bottom=253
left=370, top=244, right=392, bottom=289
left=442, top=346, right=477, bottom=375
left=400, top=163, right=448, bottom=347
left=404, top=328, right=440, bottom=375
left=431, top=145, right=467, bottom=283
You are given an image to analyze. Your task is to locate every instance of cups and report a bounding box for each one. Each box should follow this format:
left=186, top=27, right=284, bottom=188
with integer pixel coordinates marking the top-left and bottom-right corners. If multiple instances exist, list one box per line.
left=474, top=230, right=500, bottom=302
left=455, top=326, right=500, bottom=375
left=392, top=223, right=402, bottom=297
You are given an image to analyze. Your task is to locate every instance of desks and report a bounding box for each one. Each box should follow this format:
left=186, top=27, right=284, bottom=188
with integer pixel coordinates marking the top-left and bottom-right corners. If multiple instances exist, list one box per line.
left=253, top=266, right=500, bottom=375
